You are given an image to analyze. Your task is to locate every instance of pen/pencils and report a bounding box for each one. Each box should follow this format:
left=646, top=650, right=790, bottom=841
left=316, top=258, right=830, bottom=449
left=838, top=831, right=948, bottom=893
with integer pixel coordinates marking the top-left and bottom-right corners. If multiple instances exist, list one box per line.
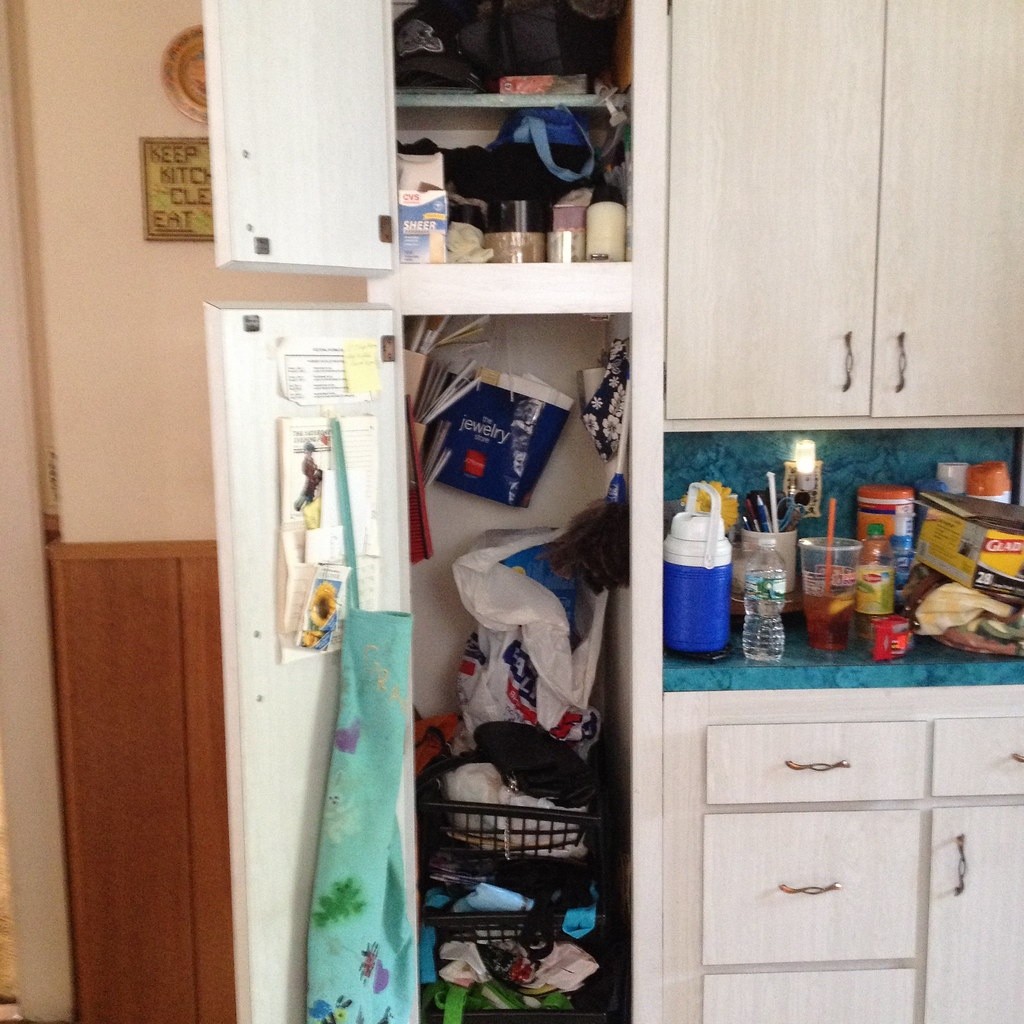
left=741, top=468, right=798, bottom=534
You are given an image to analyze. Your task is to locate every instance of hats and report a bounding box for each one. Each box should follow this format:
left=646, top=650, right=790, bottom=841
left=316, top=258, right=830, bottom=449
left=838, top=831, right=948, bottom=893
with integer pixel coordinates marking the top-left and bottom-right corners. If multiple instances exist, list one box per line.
left=581, top=336, right=628, bottom=463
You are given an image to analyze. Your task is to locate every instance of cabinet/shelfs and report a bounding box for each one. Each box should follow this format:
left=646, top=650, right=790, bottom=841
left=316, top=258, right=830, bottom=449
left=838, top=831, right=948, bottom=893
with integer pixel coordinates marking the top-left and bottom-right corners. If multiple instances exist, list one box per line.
left=198, top=0, right=1024, bottom=1024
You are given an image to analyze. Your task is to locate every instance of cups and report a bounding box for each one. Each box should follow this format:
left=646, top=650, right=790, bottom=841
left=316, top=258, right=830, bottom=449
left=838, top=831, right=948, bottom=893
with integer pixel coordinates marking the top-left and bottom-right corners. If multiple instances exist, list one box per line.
left=741, top=529, right=797, bottom=593
left=798, top=538, right=863, bottom=650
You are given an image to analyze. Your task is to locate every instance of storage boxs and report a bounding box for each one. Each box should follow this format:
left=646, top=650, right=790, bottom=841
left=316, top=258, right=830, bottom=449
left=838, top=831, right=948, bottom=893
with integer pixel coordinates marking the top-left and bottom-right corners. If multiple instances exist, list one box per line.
left=397, top=152, right=454, bottom=264
left=500, top=74, right=589, bottom=96
left=905, top=490, right=1024, bottom=612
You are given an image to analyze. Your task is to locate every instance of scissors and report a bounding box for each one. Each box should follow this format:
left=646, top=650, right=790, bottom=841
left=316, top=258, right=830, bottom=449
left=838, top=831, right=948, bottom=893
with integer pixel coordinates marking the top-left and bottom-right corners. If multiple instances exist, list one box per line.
left=776, top=498, right=805, bottom=532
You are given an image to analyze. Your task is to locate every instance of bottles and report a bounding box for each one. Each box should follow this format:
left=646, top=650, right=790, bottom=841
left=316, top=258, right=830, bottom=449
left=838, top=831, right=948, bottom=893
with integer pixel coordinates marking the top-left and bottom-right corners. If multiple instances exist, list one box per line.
left=889, top=535, right=913, bottom=612
left=664, top=483, right=732, bottom=653
left=968, top=461, right=1010, bottom=504
left=856, top=524, right=894, bottom=640
left=742, top=538, right=786, bottom=662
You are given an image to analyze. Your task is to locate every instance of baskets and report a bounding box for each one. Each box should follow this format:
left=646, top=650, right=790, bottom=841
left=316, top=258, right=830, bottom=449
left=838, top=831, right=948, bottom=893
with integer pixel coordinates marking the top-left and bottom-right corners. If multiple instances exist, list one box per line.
left=419, top=877, right=607, bottom=960
left=412, top=761, right=602, bottom=872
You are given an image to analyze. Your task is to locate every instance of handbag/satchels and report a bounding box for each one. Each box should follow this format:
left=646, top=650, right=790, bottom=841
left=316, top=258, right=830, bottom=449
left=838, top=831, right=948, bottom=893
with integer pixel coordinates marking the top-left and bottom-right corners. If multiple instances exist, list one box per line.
left=455, top=527, right=608, bottom=760
left=478, top=105, right=625, bottom=233
left=433, top=324, right=570, bottom=506
